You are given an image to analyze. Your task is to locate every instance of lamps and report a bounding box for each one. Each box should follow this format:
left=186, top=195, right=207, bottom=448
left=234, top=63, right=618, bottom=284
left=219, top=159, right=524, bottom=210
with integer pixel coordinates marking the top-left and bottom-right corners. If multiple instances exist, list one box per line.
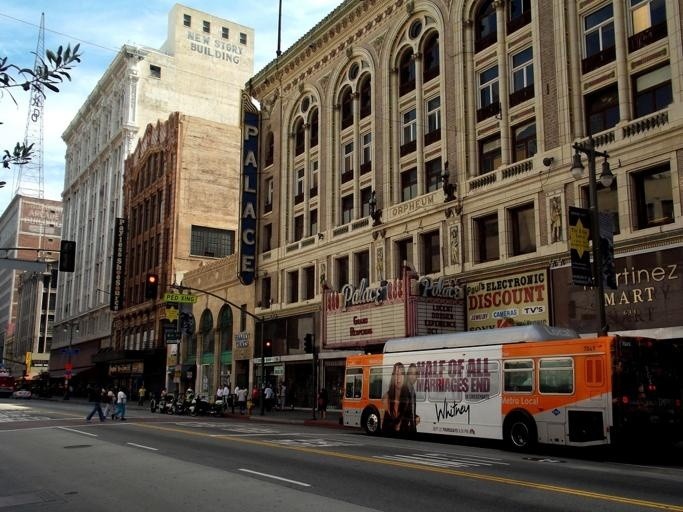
left=368, top=190, right=382, bottom=227
left=440, top=161, right=456, bottom=203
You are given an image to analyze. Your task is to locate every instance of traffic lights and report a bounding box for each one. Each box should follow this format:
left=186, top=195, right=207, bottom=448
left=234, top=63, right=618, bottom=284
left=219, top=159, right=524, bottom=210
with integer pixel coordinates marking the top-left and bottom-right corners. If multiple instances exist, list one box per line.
left=264, top=338, right=272, bottom=356
left=145, top=272, right=158, bottom=300
left=304, top=334, right=313, bottom=353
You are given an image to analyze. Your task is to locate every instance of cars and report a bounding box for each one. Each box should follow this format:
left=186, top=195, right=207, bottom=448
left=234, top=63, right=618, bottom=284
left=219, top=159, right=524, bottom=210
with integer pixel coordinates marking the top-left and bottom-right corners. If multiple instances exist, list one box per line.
left=12, top=387, right=32, bottom=400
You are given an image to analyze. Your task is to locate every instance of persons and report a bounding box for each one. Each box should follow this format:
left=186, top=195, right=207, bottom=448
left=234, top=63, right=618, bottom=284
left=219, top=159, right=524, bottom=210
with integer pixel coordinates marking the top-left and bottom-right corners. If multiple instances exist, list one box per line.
left=382, top=362, right=420, bottom=434
left=86, top=381, right=327, bottom=422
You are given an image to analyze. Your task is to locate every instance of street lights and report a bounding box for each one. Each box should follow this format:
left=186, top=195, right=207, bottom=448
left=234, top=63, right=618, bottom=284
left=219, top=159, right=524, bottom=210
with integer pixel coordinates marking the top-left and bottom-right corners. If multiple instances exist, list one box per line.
left=571, top=134, right=615, bottom=337
left=63, top=320, right=79, bottom=400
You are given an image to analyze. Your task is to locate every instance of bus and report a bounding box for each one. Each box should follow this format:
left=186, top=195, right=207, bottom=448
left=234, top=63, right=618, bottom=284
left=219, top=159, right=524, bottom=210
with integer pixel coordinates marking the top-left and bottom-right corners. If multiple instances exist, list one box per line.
left=0, top=376, right=15, bottom=398
left=342, top=324, right=683, bottom=450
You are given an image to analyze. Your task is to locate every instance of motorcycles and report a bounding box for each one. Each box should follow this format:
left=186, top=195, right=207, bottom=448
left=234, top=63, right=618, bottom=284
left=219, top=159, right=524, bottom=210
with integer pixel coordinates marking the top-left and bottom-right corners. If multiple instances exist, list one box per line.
left=159, top=394, right=227, bottom=417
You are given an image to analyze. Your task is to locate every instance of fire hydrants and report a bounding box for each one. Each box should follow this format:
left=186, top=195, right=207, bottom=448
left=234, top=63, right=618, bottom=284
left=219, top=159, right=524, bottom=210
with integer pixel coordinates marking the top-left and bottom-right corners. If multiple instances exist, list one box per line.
left=247, top=400, right=255, bottom=416
